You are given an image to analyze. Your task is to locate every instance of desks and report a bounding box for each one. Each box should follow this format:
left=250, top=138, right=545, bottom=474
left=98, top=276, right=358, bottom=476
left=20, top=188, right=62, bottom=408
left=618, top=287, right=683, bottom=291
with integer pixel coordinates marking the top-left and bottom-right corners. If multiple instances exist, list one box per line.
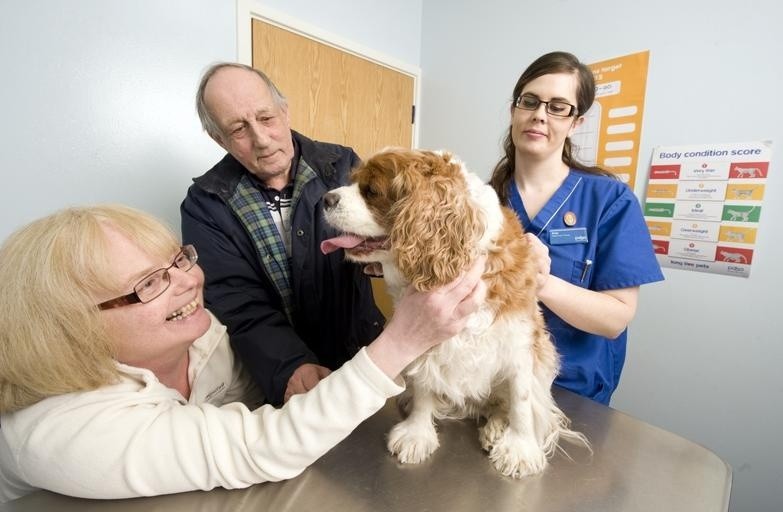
left=0, top=383, right=733, bottom=512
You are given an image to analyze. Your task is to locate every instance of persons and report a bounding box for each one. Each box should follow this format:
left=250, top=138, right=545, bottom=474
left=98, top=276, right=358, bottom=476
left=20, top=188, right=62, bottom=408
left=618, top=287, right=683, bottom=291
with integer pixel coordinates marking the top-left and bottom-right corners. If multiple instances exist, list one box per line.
left=180, top=64, right=390, bottom=403
left=0, top=204, right=486, bottom=502
left=487, top=51, right=667, bottom=408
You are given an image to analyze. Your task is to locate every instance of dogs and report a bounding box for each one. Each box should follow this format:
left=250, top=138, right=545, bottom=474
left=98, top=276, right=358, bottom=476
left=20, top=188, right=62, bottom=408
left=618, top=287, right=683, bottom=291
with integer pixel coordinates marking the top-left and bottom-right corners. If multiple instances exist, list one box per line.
left=319, top=147, right=595, bottom=481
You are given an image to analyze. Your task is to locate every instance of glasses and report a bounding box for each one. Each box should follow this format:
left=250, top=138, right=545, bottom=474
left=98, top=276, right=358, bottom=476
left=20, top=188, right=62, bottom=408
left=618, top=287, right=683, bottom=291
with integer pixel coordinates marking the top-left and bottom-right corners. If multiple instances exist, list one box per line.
left=515, top=95, right=579, bottom=117
left=93, top=244, right=199, bottom=311
